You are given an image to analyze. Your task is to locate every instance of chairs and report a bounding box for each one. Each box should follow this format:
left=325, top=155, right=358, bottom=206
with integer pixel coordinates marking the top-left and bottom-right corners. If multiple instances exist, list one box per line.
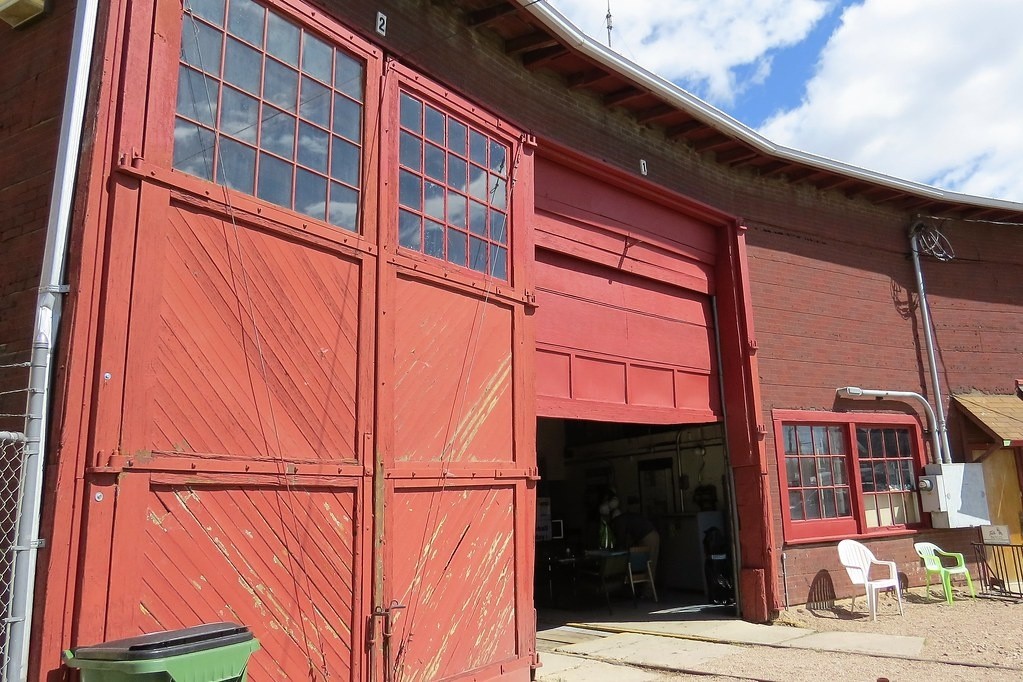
left=622, top=545, right=660, bottom=605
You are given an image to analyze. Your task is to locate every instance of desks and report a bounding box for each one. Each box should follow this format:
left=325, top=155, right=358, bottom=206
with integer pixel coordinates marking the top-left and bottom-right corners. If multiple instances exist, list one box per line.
left=580, top=550, right=628, bottom=616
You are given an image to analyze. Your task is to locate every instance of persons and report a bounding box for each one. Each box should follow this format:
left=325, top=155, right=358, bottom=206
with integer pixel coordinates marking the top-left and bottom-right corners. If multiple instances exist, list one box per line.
left=609, top=508, right=662, bottom=599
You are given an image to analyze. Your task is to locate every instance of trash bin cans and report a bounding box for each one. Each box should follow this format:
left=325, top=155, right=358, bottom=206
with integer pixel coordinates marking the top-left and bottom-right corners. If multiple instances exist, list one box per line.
left=61, top=622, right=260, bottom=682
